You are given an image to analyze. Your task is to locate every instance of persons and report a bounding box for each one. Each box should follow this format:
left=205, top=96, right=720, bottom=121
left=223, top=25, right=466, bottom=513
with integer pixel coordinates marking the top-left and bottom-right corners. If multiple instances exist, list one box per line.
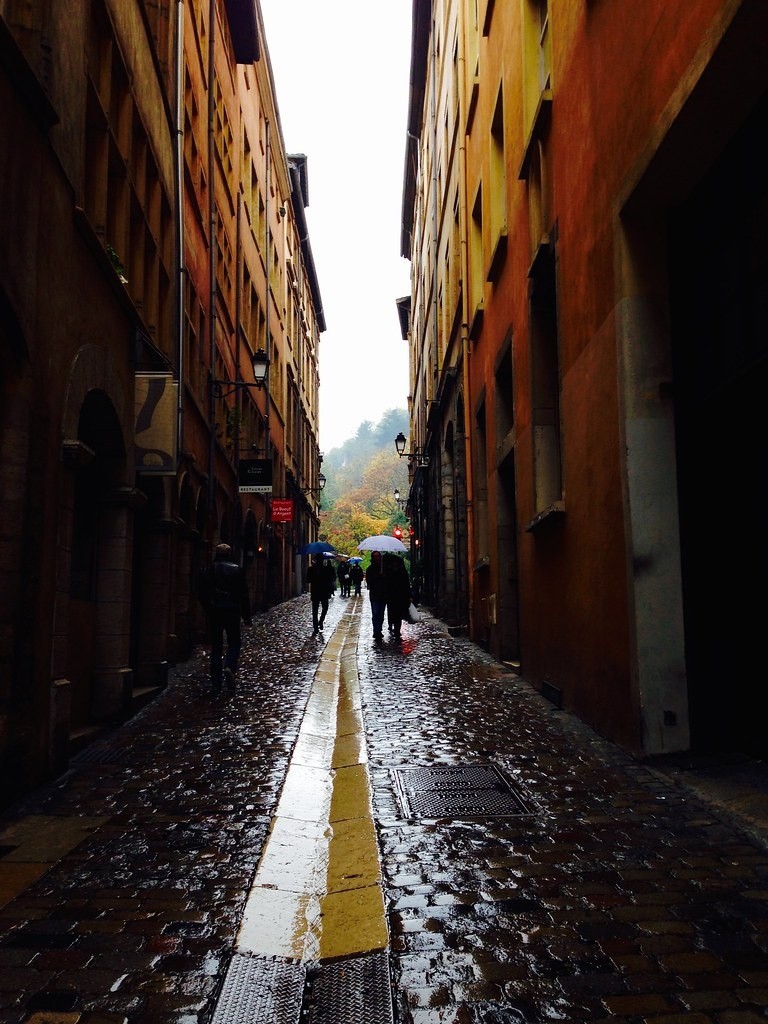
left=307, top=553, right=333, bottom=635
left=338, top=561, right=364, bottom=597
left=196, top=544, right=253, bottom=693
left=366, top=551, right=421, bottom=640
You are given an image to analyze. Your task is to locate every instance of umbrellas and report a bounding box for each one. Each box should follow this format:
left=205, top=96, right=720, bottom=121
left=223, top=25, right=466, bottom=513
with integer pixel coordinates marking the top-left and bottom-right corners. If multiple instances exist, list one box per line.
left=334, top=554, right=363, bottom=562
left=356, top=535, right=410, bottom=574
left=294, top=541, right=337, bottom=555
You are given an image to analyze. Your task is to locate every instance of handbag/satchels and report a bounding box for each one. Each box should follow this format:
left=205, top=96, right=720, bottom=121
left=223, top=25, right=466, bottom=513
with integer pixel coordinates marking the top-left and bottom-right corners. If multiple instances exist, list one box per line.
left=344, top=574, right=349, bottom=579
left=409, top=602, right=420, bottom=624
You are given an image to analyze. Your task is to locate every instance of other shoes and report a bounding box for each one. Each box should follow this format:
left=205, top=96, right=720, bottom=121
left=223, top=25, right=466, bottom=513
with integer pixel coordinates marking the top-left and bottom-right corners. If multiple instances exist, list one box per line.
left=224, top=666, right=236, bottom=691
left=209, top=693, right=226, bottom=702
left=319, top=620, right=323, bottom=630
left=314, top=629, right=319, bottom=633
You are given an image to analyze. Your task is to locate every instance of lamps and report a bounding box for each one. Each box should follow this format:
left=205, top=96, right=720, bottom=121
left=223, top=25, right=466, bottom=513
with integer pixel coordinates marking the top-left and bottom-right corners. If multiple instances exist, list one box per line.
left=207, top=347, right=271, bottom=391
left=301, top=473, right=326, bottom=489
left=393, top=489, right=410, bottom=502
left=395, top=431, right=431, bottom=465
